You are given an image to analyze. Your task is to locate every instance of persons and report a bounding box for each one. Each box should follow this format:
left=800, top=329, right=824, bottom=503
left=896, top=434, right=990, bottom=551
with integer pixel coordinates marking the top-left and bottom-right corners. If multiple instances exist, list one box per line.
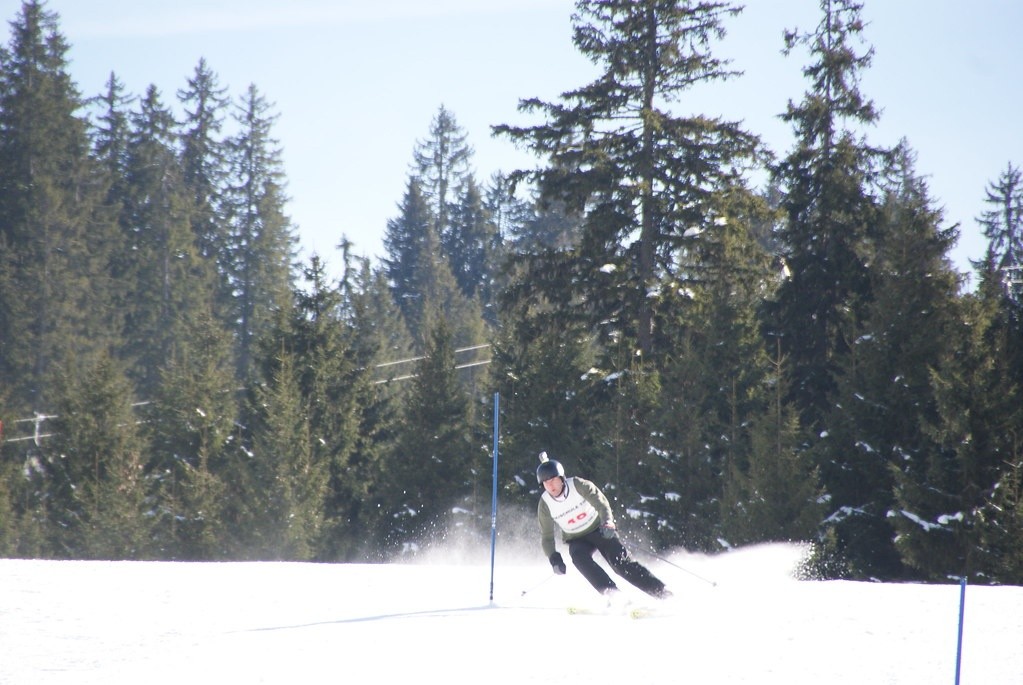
left=534, top=459, right=673, bottom=600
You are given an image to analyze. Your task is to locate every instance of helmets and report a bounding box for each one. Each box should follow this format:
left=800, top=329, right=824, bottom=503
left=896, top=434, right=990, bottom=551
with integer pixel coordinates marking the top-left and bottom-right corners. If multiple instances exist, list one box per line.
left=536, top=459, right=565, bottom=484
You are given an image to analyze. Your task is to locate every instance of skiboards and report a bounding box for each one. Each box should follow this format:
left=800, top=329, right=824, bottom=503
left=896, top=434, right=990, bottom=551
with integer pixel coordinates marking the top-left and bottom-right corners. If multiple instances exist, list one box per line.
left=564, top=605, right=645, bottom=620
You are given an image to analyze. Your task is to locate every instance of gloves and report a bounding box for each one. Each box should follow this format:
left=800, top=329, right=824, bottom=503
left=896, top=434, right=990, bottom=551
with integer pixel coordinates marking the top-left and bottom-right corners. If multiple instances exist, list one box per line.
left=600, top=520, right=615, bottom=540
left=549, top=551, right=566, bottom=575
